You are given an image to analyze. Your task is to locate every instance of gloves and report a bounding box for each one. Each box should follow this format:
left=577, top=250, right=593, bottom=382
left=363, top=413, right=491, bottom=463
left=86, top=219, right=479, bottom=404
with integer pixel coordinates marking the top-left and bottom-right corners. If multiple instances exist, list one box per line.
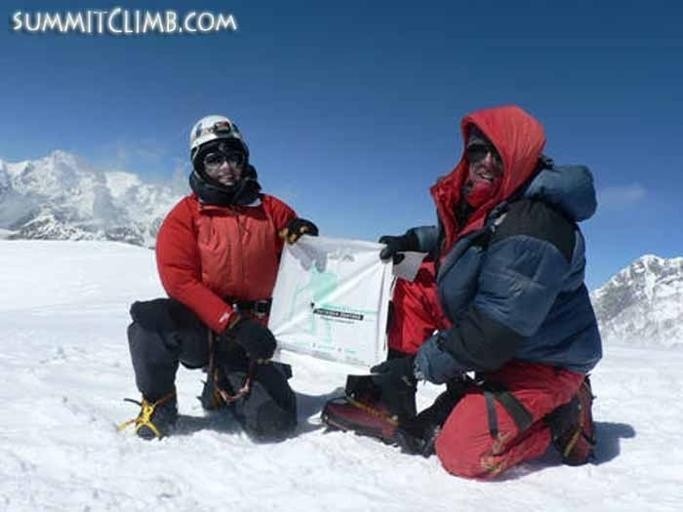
left=370, top=354, right=419, bottom=396
left=378, top=226, right=422, bottom=265
left=223, top=316, right=278, bottom=361
left=277, top=214, right=320, bottom=245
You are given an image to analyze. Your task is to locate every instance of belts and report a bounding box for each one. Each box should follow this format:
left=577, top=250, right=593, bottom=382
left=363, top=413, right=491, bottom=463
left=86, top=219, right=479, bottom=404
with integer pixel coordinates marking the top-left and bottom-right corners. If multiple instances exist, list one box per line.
left=226, top=298, right=275, bottom=320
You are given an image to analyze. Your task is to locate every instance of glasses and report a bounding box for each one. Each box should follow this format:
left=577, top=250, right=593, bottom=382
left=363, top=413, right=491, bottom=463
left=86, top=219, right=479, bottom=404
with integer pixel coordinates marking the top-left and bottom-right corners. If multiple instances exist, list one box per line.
left=463, top=141, right=503, bottom=165
left=202, top=152, right=247, bottom=169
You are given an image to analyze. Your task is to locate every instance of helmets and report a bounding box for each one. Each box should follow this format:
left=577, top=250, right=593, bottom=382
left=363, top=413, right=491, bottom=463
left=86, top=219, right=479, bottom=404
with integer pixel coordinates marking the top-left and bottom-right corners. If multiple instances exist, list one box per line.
left=189, top=113, right=250, bottom=154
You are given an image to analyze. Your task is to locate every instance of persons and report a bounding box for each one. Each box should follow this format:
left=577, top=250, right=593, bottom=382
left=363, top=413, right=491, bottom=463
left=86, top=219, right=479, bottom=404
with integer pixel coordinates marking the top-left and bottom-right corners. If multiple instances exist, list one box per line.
left=323, top=104, right=602, bottom=479
left=127, top=112, right=320, bottom=440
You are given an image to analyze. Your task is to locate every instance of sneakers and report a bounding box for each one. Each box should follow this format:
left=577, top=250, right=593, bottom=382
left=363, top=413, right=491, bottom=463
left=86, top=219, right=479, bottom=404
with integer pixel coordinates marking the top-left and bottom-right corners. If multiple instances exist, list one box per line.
left=552, top=373, right=600, bottom=467
left=134, top=388, right=177, bottom=440
left=197, top=371, right=228, bottom=413
left=318, top=391, right=401, bottom=446
